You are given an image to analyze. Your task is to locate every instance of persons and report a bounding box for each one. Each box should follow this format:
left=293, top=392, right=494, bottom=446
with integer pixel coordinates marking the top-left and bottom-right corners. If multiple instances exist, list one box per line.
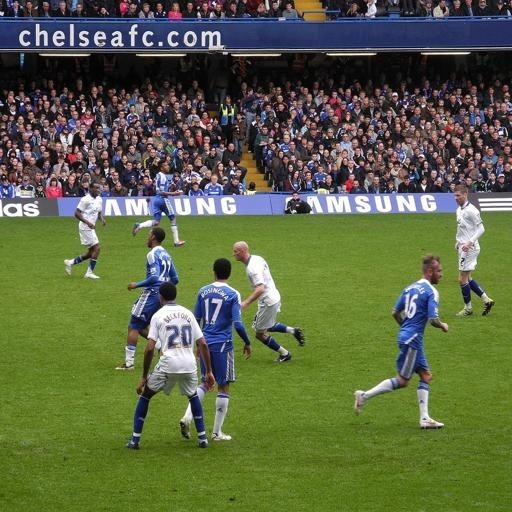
left=453, top=185, right=494, bottom=318
left=353, top=253, right=448, bottom=429
left=123, top=283, right=216, bottom=451
left=1, top=1, right=512, bottom=198
left=177, top=258, right=252, bottom=440
left=285, top=192, right=312, bottom=215
left=233, top=240, right=305, bottom=361
left=114, top=226, right=179, bottom=371
left=64, top=181, right=106, bottom=279
left=131, top=160, right=186, bottom=245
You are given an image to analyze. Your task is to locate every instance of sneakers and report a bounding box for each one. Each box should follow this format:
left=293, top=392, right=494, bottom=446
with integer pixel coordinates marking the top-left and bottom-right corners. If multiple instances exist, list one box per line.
left=353, top=389, right=369, bottom=415
left=132, top=223, right=140, bottom=237
left=293, top=326, right=305, bottom=346
left=180, top=418, right=232, bottom=447
left=174, top=240, right=185, bottom=247
left=273, top=352, right=291, bottom=363
left=419, top=418, right=444, bottom=430
left=64, top=260, right=71, bottom=277
left=125, top=439, right=140, bottom=449
left=482, top=297, right=495, bottom=317
left=84, top=274, right=99, bottom=279
left=455, top=306, right=473, bottom=318
left=115, top=363, right=135, bottom=371
